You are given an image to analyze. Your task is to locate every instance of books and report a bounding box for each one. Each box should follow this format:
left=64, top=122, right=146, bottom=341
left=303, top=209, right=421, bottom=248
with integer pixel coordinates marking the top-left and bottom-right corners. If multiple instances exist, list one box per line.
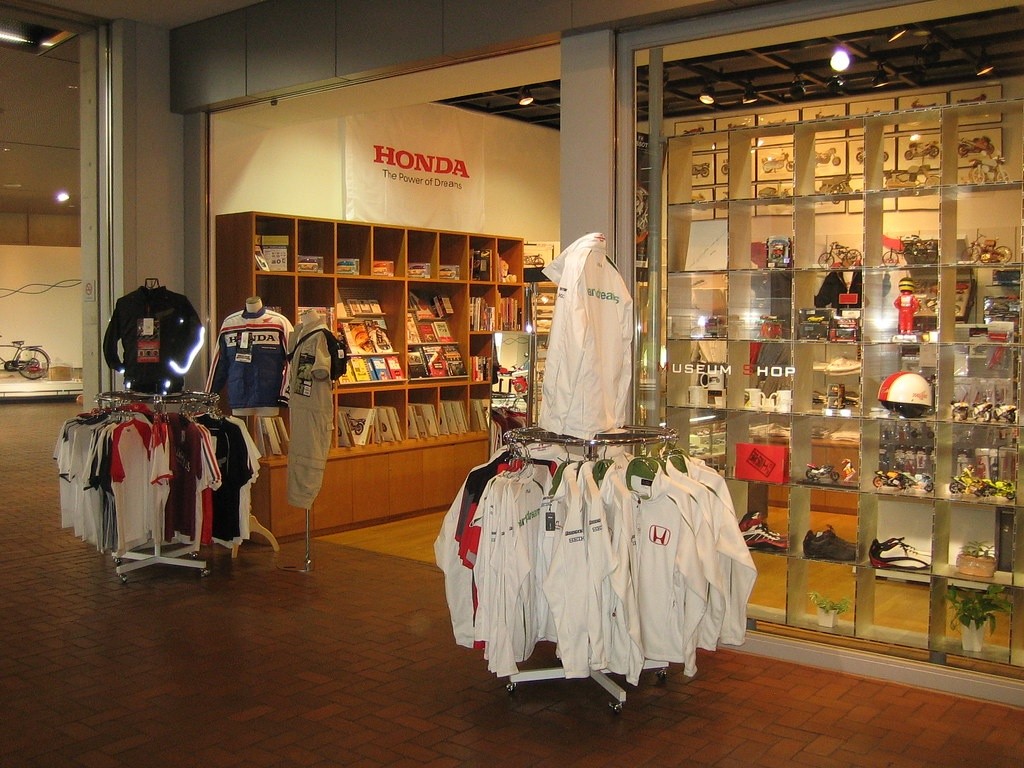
left=497, top=290, right=523, bottom=331
left=468, top=249, right=492, bottom=282
left=469, top=298, right=496, bottom=332
left=469, top=356, right=492, bottom=382
left=407, top=292, right=467, bottom=378
left=336, top=299, right=404, bottom=385
left=254, top=417, right=289, bottom=457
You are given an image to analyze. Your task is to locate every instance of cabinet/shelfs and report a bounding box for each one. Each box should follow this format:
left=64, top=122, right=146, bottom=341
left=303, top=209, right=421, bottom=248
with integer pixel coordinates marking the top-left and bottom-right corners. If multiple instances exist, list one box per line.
left=665, top=95, right=1024, bottom=680
left=768, top=434, right=860, bottom=517
left=214, top=211, right=526, bottom=547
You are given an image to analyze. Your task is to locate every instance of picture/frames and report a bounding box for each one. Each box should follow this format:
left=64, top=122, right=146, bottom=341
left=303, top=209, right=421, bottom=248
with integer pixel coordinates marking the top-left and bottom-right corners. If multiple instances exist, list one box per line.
left=691, top=126, right=1005, bottom=222
left=757, top=108, right=801, bottom=147
left=715, top=114, right=757, bottom=151
left=897, top=92, right=948, bottom=133
left=848, top=98, right=896, bottom=138
left=801, top=103, right=847, bottom=140
left=674, top=119, right=715, bottom=153
left=949, top=84, right=1004, bottom=127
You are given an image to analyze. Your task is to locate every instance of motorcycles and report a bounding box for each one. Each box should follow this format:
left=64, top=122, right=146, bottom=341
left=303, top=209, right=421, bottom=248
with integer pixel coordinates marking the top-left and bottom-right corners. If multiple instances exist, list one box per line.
left=873, top=470, right=933, bottom=492
left=948, top=465, right=1016, bottom=500
left=950, top=394, right=1017, bottom=423
left=806, top=463, right=840, bottom=482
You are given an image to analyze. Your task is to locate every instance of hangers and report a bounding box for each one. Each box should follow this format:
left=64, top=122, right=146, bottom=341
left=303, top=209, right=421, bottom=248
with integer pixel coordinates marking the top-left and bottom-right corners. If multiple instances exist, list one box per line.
left=90, top=390, right=230, bottom=424
left=502, top=425, right=688, bottom=497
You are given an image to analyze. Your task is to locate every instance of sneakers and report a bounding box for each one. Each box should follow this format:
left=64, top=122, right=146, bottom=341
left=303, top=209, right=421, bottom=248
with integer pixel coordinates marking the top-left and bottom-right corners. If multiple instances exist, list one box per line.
left=803, top=527, right=856, bottom=561
left=738, top=510, right=787, bottom=552
left=869, top=537, right=933, bottom=570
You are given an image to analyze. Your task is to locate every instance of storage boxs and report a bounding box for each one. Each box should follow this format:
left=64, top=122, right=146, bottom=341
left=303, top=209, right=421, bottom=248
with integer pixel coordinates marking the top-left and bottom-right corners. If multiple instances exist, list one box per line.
left=735, top=441, right=792, bottom=484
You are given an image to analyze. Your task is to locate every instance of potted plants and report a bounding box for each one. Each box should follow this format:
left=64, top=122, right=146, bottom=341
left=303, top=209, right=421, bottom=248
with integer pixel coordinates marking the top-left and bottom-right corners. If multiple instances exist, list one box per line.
left=806, top=590, right=853, bottom=628
left=942, top=583, right=1012, bottom=652
left=955, top=540, right=999, bottom=577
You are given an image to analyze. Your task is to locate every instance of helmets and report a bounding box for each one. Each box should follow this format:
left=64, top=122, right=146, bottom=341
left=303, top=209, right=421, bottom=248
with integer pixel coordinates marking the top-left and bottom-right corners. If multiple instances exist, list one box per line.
left=878, top=371, right=931, bottom=408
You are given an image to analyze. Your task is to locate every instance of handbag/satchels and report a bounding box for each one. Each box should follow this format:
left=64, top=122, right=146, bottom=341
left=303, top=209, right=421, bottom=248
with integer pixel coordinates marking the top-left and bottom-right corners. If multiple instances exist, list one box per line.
left=294, top=329, right=347, bottom=381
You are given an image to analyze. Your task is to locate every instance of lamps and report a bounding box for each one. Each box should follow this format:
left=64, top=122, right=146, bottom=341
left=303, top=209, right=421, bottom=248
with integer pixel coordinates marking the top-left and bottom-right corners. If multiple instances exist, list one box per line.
left=969, top=53, right=996, bottom=76
left=518, top=86, right=534, bottom=106
left=871, top=71, right=888, bottom=88
left=788, top=81, right=806, bottom=100
left=742, top=91, right=758, bottom=104
left=699, top=86, right=716, bottom=104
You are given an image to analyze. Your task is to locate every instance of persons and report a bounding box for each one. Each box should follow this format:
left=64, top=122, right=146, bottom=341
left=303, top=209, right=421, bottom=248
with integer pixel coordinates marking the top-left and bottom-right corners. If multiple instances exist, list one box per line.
left=103, top=277, right=202, bottom=396
left=287, top=308, right=331, bottom=508
left=205, top=296, right=295, bottom=416
left=814, top=256, right=862, bottom=308
left=894, top=277, right=919, bottom=335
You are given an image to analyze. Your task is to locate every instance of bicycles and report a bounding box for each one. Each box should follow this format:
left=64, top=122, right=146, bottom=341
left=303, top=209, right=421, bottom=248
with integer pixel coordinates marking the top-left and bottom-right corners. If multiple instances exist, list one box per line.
left=818, top=241, right=862, bottom=268
left=881, top=235, right=929, bottom=266
left=0, top=334, right=51, bottom=380
left=961, top=228, right=1012, bottom=264
